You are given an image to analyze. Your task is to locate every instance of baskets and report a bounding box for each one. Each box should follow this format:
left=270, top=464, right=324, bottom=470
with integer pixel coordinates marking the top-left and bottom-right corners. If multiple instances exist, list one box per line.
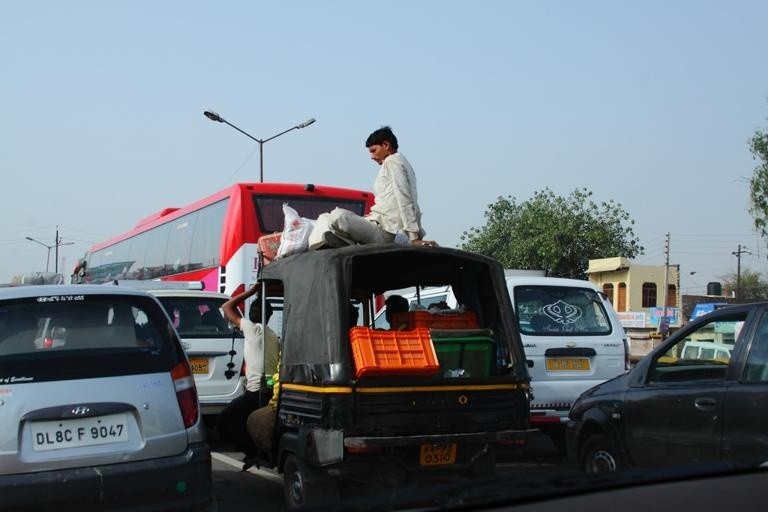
left=348, top=326, right=441, bottom=381
left=407, top=311, right=481, bottom=332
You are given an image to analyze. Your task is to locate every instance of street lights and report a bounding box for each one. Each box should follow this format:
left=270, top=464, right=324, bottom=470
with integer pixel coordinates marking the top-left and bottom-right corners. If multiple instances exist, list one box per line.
left=26, top=236, right=73, bottom=270
left=204, top=110, right=315, bottom=183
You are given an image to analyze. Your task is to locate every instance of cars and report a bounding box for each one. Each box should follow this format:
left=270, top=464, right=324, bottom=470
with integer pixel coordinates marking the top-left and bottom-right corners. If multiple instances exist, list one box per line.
left=565, top=299, right=767, bottom=486
left=145, top=290, right=259, bottom=418
left=1, top=284, right=215, bottom=511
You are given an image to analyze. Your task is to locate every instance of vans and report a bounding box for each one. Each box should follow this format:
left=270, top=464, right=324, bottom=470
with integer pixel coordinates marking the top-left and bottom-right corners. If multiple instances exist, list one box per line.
left=366, top=276, right=633, bottom=414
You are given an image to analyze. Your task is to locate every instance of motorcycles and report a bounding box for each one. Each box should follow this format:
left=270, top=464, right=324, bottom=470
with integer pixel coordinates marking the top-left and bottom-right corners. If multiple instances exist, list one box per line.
left=242, top=243, right=540, bottom=508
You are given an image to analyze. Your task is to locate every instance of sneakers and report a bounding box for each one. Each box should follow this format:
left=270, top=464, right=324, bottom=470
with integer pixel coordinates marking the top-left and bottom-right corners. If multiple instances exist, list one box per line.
left=324, top=230, right=355, bottom=247
left=242, top=447, right=262, bottom=472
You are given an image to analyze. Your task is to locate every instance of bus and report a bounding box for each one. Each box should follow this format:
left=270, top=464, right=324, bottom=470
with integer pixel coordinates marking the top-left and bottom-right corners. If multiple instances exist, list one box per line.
left=71, top=181, right=386, bottom=343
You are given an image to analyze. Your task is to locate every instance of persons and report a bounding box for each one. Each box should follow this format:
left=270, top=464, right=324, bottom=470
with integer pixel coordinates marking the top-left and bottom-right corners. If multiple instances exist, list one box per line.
left=221, top=283, right=280, bottom=473
left=375, top=296, right=409, bottom=332
left=308, top=126, right=438, bottom=247
